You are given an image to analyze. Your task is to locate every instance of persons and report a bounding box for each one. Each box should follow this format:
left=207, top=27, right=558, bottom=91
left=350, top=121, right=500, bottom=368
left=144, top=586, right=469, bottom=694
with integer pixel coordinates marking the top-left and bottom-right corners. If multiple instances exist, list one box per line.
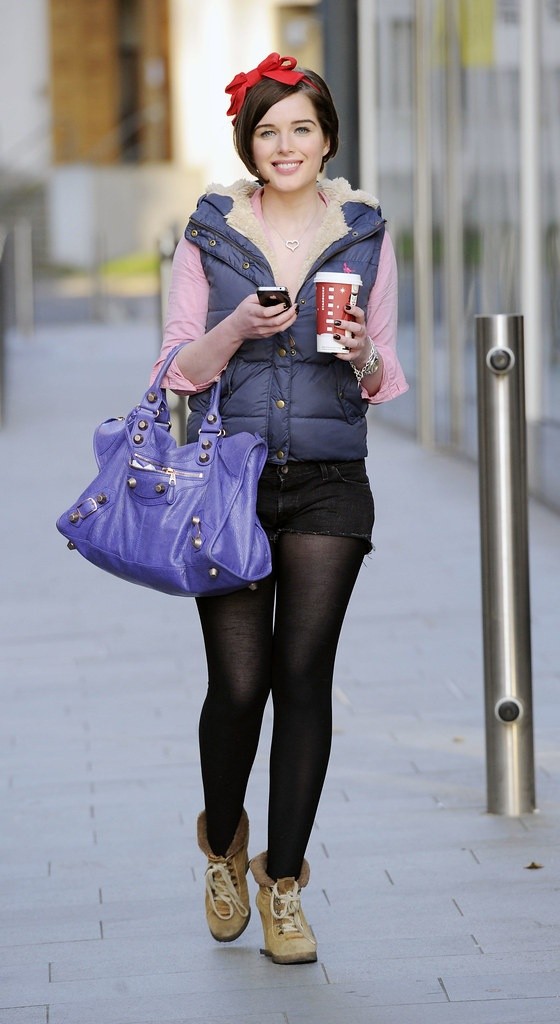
left=150, top=52, right=409, bottom=963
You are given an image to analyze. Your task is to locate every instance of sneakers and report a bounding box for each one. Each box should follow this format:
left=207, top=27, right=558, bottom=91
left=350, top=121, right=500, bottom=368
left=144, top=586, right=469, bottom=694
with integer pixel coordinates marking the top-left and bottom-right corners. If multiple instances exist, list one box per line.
left=247, top=846, right=316, bottom=966
left=194, top=809, right=252, bottom=942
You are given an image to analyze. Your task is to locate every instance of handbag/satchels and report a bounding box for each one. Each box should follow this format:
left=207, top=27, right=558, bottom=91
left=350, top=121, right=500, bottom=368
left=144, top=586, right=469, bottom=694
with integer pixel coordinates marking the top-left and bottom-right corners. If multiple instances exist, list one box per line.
left=54, top=338, right=275, bottom=597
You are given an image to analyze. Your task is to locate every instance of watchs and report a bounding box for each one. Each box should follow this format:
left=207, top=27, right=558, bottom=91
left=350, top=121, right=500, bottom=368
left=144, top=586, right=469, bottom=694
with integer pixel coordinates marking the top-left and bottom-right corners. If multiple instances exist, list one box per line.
left=364, top=347, right=380, bottom=376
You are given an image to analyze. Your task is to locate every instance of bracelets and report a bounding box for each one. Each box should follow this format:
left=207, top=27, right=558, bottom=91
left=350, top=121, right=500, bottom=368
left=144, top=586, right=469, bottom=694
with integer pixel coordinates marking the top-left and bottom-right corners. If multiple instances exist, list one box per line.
left=349, top=335, right=375, bottom=389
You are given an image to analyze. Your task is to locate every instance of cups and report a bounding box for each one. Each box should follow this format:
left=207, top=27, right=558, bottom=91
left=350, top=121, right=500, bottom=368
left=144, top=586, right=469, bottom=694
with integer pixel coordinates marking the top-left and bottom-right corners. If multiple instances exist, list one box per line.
left=313, top=271, right=364, bottom=355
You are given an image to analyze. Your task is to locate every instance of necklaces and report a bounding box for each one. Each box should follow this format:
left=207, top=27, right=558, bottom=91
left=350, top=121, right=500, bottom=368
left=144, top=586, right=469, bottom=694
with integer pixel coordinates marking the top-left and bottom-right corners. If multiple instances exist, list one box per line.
left=261, top=193, right=320, bottom=253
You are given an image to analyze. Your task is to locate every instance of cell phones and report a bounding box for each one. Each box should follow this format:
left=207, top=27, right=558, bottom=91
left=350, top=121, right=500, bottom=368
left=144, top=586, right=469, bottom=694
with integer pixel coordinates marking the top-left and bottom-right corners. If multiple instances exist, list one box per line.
left=256, top=287, right=292, bottom=317
